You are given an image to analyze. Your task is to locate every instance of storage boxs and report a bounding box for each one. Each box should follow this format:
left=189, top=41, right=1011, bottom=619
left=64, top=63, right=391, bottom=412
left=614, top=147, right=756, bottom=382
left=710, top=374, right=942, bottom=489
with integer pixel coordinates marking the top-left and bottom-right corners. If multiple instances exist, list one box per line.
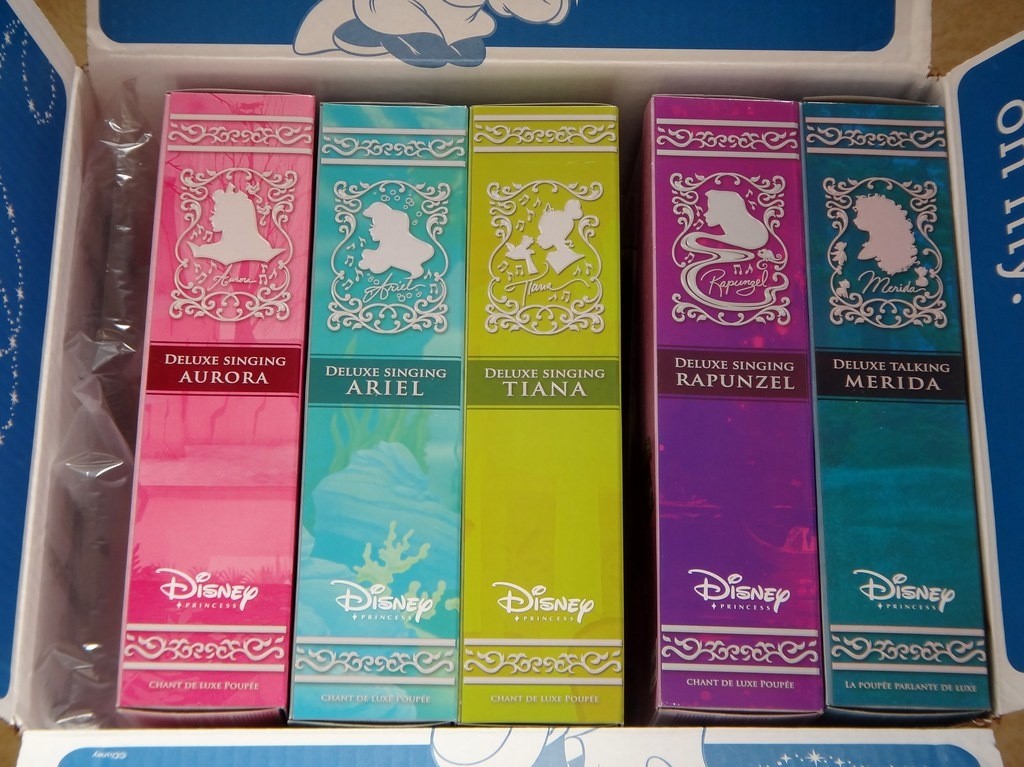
left=0, top=0, right=1024, bottom=767
